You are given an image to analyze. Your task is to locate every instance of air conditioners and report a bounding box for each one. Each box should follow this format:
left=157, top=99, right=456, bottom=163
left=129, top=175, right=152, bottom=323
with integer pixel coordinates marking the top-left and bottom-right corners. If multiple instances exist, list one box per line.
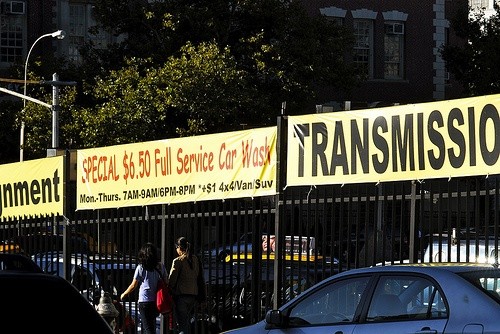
left=388, top=23, right=404, bottom=34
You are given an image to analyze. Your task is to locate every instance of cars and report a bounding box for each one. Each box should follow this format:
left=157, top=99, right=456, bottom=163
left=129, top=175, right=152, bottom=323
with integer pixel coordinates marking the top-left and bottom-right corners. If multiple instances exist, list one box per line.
left=218, top=262, right=500, bottom=334
left=0, top=239, right=218, bottom=334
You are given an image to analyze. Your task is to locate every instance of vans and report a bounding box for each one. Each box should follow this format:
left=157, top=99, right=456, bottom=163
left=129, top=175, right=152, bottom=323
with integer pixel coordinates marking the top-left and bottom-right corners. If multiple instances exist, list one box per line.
left=421, top=239, right=500, bottom=296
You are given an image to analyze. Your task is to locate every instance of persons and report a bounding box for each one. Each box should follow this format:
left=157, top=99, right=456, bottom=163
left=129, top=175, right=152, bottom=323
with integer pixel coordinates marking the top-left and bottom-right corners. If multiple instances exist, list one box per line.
left=167, top=237, right=200, bottom=330
left=120, top=244, right=166, bottom=334
left=262, top=235, right=276, bottom=253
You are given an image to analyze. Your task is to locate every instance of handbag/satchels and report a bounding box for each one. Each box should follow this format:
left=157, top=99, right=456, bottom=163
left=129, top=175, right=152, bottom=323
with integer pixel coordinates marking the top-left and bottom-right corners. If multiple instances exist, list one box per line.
left=155, top=263, right=173, bottom=314
left=196, top=255, right=206, bottom=303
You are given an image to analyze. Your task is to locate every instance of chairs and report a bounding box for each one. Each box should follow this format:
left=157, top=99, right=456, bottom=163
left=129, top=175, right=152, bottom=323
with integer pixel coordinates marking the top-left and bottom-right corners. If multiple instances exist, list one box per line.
left=374, top=293, right=403, bottom=317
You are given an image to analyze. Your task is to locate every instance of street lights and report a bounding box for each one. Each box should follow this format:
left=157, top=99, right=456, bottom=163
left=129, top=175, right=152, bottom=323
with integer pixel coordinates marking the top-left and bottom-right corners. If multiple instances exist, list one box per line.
left=19, top=29, right=66, bottom=163
